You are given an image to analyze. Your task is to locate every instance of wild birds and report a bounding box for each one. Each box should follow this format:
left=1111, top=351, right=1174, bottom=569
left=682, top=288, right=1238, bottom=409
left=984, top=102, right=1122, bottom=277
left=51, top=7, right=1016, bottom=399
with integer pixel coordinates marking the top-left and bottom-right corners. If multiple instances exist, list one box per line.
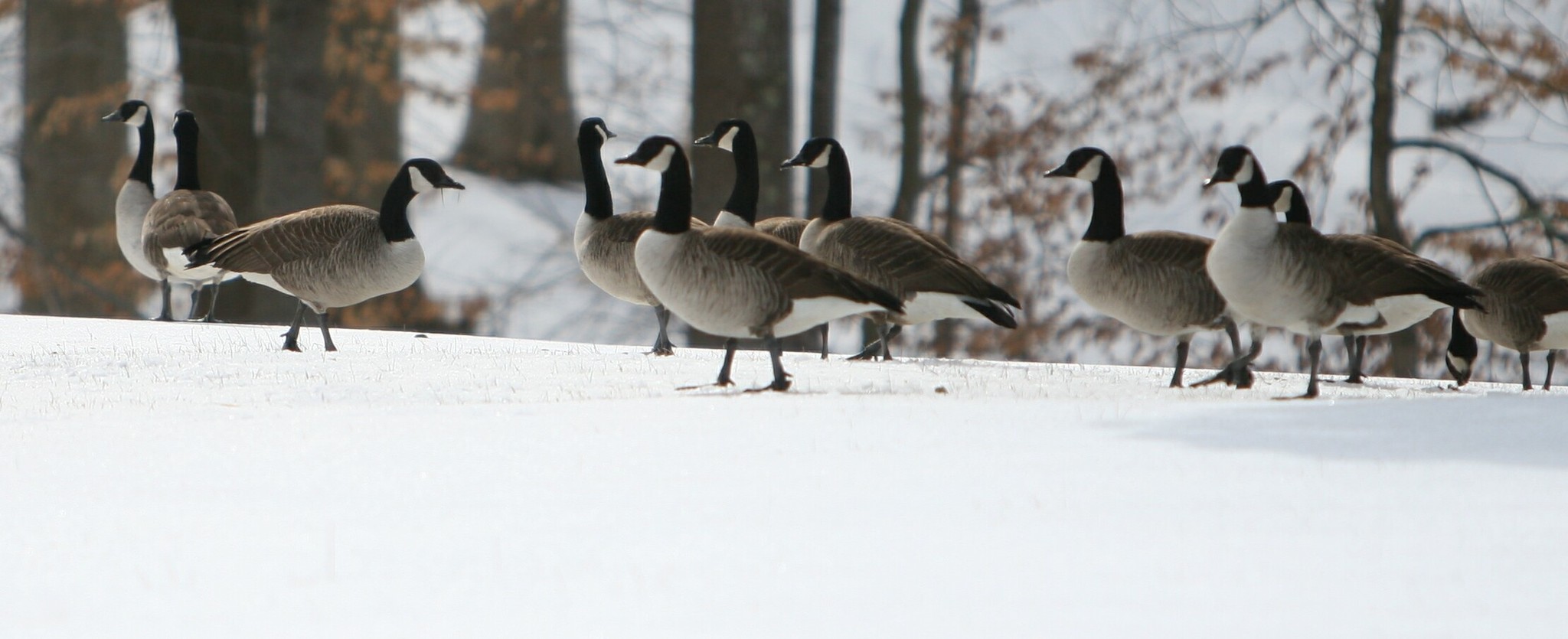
left=101, top=99, right=238, bottom=324
left=1460, top=256, right=1568, bottom=392
left=573, top=117, right=712, bottom=357
left=1202, top=143, right=1490, bottom=403
left=1042, top=147, right=1256, bottom=391
left=613, top=135, right=907, bottom=394
left=180, top=158, right=466, bottom=352
left=693, top=118, right=1023, bottom=364
